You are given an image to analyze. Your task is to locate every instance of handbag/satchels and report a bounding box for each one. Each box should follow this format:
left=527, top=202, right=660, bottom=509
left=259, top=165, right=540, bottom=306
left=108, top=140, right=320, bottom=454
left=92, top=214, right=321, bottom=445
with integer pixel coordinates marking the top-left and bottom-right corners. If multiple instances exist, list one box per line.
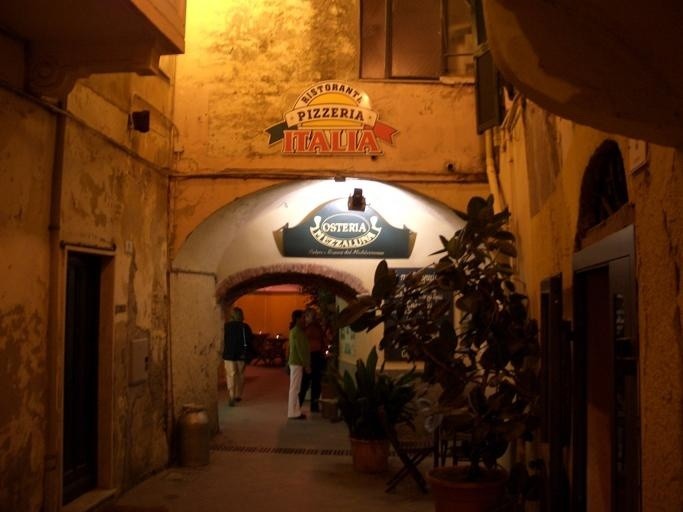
left=243, top=345, right=254, bottom=365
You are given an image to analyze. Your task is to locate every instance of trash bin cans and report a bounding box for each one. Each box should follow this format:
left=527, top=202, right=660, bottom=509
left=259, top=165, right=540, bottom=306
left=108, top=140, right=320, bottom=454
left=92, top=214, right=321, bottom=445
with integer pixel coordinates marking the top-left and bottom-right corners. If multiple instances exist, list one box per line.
left=173, top=403, right=211, bottom=468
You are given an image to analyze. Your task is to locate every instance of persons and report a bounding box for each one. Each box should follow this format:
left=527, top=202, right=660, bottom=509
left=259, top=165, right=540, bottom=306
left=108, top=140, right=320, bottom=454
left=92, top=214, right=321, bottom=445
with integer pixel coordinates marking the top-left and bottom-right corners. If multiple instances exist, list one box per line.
left=221, top=306, right=253, bottom=407
left=285, top=309, right=311, bottom=421
left=299, top=306, right=327, bottom=419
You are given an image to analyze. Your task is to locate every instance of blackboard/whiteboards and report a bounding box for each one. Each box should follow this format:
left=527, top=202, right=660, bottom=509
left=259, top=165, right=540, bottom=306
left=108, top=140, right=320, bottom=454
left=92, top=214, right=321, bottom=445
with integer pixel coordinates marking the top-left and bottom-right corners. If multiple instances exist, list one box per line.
left=384, top=267, right=453, bottom=361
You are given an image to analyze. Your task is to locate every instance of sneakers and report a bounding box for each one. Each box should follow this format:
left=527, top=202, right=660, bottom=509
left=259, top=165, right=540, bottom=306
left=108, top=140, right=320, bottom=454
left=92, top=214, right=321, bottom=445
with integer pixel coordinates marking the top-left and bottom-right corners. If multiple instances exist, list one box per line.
left=228, top=397, right=242, bottom=407
left=287, top=413, right=306, bottom=420
